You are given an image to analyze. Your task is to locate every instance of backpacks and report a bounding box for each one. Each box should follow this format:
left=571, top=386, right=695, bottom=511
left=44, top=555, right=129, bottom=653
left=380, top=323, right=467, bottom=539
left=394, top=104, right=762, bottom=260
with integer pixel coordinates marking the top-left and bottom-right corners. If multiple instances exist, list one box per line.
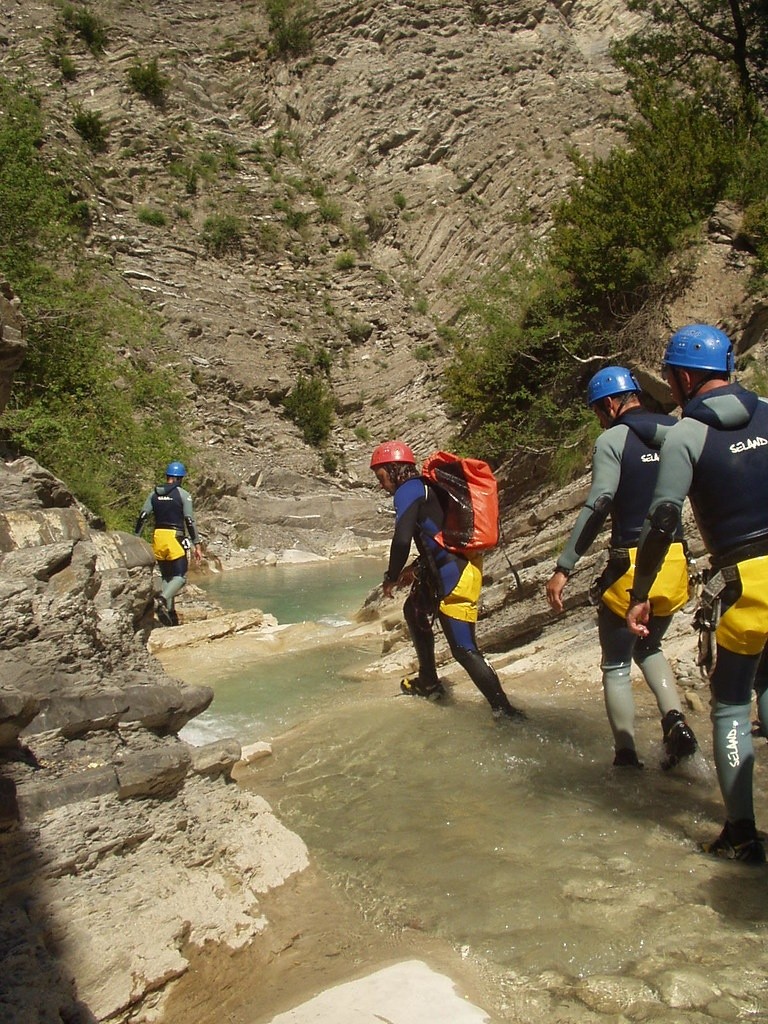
left=400, top=451, right=499, bottom=557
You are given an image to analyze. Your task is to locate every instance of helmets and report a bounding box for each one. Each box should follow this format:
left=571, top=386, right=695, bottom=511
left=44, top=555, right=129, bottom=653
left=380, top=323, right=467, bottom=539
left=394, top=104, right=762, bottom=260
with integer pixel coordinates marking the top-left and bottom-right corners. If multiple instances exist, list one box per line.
left=663, top=323, right=736, bottom=373
left=165, top=462, right=187, bottom=476
left=587, top=366, right=642, bottom=406
left=370, top=441, right=418, bottom=467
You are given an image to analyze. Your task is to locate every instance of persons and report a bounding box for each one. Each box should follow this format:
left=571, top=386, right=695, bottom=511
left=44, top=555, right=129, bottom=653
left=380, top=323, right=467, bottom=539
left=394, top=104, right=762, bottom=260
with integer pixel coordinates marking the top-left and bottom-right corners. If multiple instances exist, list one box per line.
left=626, top=324, right=768, bottom=857
left=134, top=463, right=202, bottom=627
left=372, top=442, right=513, bottom=715
left=545, top=366, right=695, bottom=768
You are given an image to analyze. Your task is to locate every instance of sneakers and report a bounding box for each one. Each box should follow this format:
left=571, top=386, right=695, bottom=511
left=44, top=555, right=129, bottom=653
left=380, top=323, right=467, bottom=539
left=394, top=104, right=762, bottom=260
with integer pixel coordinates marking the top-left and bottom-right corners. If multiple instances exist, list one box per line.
left=696, top=822, right=766, bottom=866
left=169, top=606, right=179, bottom=626
left=154, top=596, right=172, bottom=627
left=660, top=710, right=698, bottom=770
left=491, top=707, right=526, bottom=728
left=613, top=750, right=645, bottom=770
left=400, top=677, right=445, bottom=701
